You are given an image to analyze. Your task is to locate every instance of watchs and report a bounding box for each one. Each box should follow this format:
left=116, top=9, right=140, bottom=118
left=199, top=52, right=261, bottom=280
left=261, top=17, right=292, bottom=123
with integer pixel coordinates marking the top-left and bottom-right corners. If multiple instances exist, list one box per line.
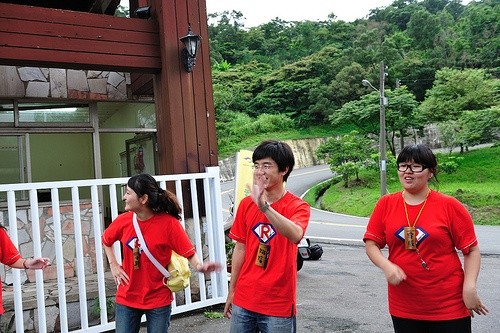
left=258, top=200, right=269, bottom=213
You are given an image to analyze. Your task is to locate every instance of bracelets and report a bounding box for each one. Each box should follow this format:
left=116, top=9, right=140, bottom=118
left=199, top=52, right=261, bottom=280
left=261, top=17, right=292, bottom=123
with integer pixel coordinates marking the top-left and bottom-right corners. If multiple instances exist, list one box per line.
left=23, top=256, right=29, bottom=270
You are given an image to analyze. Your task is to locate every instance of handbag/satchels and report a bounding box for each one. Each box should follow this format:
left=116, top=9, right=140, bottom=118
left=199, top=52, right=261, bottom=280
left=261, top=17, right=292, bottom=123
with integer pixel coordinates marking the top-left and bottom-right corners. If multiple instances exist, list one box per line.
left=163, top=250, right=191, bottom=292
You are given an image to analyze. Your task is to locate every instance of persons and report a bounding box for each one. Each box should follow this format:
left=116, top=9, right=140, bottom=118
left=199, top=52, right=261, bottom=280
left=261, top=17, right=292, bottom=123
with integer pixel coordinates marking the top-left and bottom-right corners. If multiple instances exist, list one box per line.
left=101, top=174, right=220, bottom=333
left=223, top=140, right=311, bottom=333
left=1, top=223, right=52, bottom=317
left=363, top=145, right=489, bottom=333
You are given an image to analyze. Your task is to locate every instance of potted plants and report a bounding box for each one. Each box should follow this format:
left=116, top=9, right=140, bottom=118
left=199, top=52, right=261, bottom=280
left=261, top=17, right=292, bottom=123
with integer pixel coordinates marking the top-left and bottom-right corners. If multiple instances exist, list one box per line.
left=225, top=242, right=236, bottom=273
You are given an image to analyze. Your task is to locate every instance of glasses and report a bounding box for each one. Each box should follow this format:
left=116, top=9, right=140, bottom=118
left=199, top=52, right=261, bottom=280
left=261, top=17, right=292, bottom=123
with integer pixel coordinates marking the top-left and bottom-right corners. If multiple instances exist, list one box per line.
left=252, top=163, right=278, bottom=171
left=396, top=163, right=428, bottom=173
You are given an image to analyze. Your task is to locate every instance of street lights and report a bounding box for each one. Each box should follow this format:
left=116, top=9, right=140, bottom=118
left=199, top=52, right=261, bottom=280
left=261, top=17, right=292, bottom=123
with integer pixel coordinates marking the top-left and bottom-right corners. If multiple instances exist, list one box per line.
left=361, top=61, right=388, bottom=197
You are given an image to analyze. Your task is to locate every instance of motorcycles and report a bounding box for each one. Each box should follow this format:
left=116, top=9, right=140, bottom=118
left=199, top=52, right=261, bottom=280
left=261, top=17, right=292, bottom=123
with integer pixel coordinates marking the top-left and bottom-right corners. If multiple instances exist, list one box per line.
left=223, top=193, right=324, bottom=272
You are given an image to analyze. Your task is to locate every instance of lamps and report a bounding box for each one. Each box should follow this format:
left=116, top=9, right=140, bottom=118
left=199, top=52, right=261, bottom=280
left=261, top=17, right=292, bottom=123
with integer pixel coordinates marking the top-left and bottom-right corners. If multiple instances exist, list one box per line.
left=179, top=23, right=203, bottom=71
